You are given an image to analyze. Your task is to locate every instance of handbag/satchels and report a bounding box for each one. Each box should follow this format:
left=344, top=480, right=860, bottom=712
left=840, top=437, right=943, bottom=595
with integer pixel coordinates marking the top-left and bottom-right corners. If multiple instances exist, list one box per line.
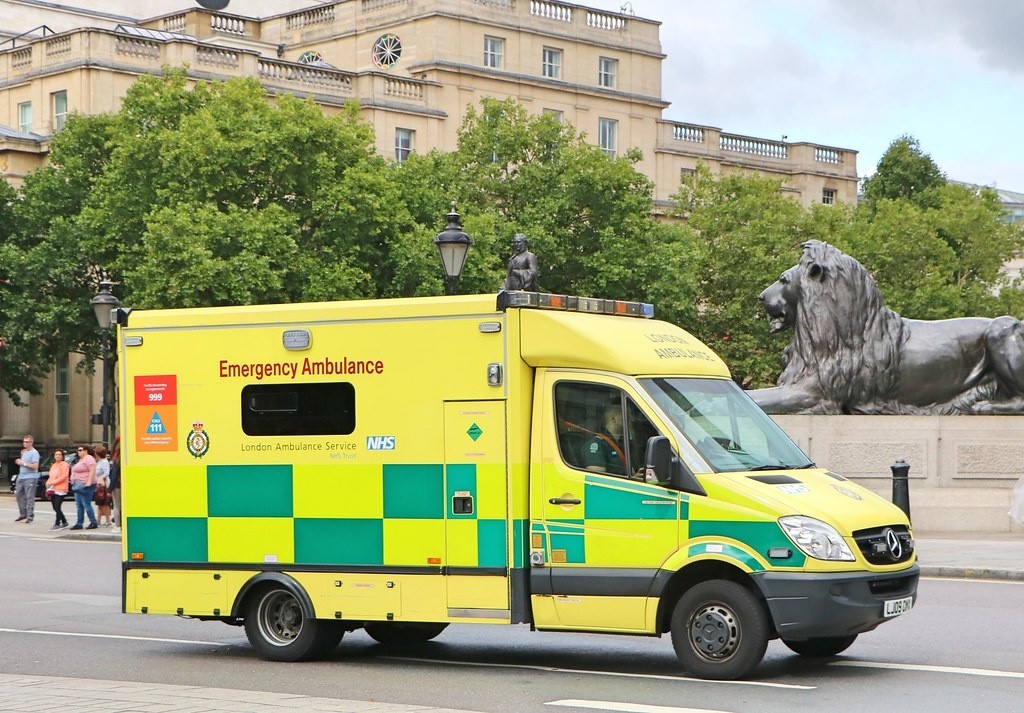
left=46, top=485, right=55, bottom=497
left=72, top=481, right=89, bottom=494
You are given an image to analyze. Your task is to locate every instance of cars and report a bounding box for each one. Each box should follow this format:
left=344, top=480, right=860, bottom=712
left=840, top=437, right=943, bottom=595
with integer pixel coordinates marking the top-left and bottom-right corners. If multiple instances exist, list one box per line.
left=10, top=448, right=97, bottom=502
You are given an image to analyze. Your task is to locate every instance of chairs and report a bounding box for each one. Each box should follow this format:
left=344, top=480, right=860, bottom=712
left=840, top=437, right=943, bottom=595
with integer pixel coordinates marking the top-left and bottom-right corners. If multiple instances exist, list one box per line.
left=558, top=402, right=590, bottom=468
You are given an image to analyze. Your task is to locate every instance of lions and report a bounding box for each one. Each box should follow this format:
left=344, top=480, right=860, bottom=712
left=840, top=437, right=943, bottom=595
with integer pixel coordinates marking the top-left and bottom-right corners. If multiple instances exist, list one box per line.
left=741, top=239, right=1024, bottom=416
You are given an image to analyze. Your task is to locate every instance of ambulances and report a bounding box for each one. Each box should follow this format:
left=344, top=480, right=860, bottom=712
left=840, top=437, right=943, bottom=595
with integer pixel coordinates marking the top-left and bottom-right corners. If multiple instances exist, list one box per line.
left=116, top=288, right=921, bottom=679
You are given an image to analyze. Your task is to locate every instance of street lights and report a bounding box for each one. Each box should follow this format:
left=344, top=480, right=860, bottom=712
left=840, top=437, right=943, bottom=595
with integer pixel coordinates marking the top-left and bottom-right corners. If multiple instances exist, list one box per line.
left=87, top=270, right=119, bottom=451
left=433, top=201, right=472, bottom=295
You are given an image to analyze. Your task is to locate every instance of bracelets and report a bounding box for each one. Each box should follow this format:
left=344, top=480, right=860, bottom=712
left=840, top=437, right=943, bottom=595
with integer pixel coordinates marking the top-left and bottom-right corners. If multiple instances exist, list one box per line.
left=24, top=462, right=27, bottom=466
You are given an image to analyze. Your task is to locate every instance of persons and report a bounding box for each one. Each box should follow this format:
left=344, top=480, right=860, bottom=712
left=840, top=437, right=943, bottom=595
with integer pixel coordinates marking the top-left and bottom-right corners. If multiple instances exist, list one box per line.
left=46, top=435, right=122, bottom=533
left=505, top=234, right=540, bottom=292
left=15, top=435, right=40, bottom=523
left=582, top=397, right=644, bottom=477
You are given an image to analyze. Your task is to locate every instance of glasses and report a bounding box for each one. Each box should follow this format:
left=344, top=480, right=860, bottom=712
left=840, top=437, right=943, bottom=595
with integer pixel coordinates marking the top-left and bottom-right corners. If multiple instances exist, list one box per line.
left=23, top=441, right=29, bottom=443
left=78, top=449, right=82, bottom=451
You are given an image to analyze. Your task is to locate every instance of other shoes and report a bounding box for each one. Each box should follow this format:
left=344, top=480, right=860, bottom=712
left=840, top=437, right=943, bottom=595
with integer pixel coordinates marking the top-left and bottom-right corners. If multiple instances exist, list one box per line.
left=96, top=522, right=100, bottom=525
left=26, top=518, right=33, bottom=523
left=15, top=516, right=26, bottom=521
left=86, top=524, right=97, bottom=529
left=70, top=524, right=83, bottom=529
left=102, top=522, right=112, bottom=527
left=48, top=522, right=69, bottom=532
left=111, top=527, right=120, bottom=532
